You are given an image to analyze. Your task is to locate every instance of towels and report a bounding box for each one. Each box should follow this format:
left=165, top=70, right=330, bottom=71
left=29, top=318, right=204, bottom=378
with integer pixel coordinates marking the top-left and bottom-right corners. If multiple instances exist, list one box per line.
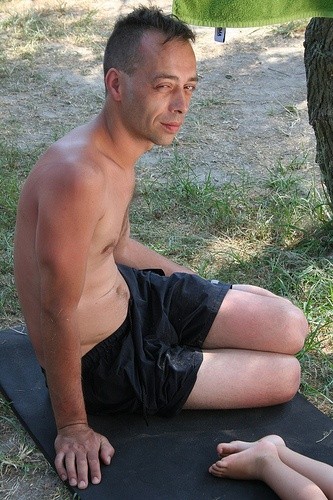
left=172, top=0, right=333, bottom=44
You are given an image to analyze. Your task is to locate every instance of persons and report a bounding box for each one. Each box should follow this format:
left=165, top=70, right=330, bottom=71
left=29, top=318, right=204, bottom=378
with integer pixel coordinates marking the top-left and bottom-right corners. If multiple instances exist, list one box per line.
left=209, top=434, right=333, bottom=499
left=13, top=2, right=309, bottom=489
